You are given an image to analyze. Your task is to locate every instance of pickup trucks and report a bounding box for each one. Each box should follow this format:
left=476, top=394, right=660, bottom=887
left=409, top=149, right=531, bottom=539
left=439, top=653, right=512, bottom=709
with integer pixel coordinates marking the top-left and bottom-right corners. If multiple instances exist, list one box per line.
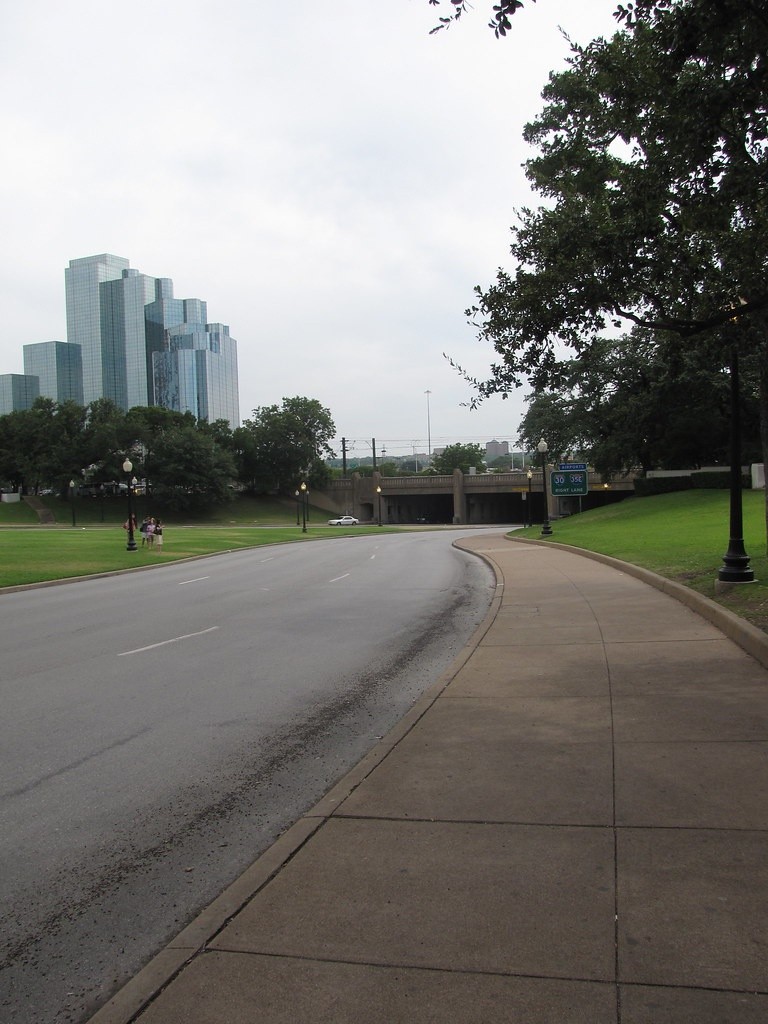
left=372, top=449, right=386, bottom=471
left=416, top=513, right=433, bottom=523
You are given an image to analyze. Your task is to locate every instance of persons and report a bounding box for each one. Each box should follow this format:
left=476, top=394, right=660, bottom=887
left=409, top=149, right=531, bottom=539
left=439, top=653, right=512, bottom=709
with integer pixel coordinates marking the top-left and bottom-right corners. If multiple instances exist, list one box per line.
left=154, top=519, right=165, bottom=554
left=124, top=512, right=138, bottom=543
left=141, top=514, right=156, bottom=551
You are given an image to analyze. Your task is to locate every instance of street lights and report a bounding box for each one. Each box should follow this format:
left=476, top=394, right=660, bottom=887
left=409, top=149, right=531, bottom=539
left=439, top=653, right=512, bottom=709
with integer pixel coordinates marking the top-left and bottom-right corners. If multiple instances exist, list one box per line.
left=537, top=436, right=554, bottom=534
left=295, top=490, right=300, bottom=525
left=306, top=490, right=309, bottom=521
left=603, top=483, right=609, bottom=506
left=122, top=457, right=138, bottom=551
left=526, top=469, right=534, bottom=527
left=132, top=476, right=137, bottom=528
left=300, top=481, right=306, bottom=533
left=424, top=389, right=432, bottom=472
left=100, top=484, right=104, bottom=522
left=376, top=485, right=383, bottom=527
left=70, top=479, right=77, bottom=527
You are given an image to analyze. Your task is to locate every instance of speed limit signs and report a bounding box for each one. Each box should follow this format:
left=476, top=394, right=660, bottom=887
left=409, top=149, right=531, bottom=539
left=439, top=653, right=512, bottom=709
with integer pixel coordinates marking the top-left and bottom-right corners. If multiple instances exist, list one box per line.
left=549, top=468, right=589, bottom=497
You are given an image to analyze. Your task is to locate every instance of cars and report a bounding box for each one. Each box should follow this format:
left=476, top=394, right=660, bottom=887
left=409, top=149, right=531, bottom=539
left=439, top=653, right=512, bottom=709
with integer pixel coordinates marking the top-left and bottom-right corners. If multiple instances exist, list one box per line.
left=328, top=515, right=360, bottom=526
